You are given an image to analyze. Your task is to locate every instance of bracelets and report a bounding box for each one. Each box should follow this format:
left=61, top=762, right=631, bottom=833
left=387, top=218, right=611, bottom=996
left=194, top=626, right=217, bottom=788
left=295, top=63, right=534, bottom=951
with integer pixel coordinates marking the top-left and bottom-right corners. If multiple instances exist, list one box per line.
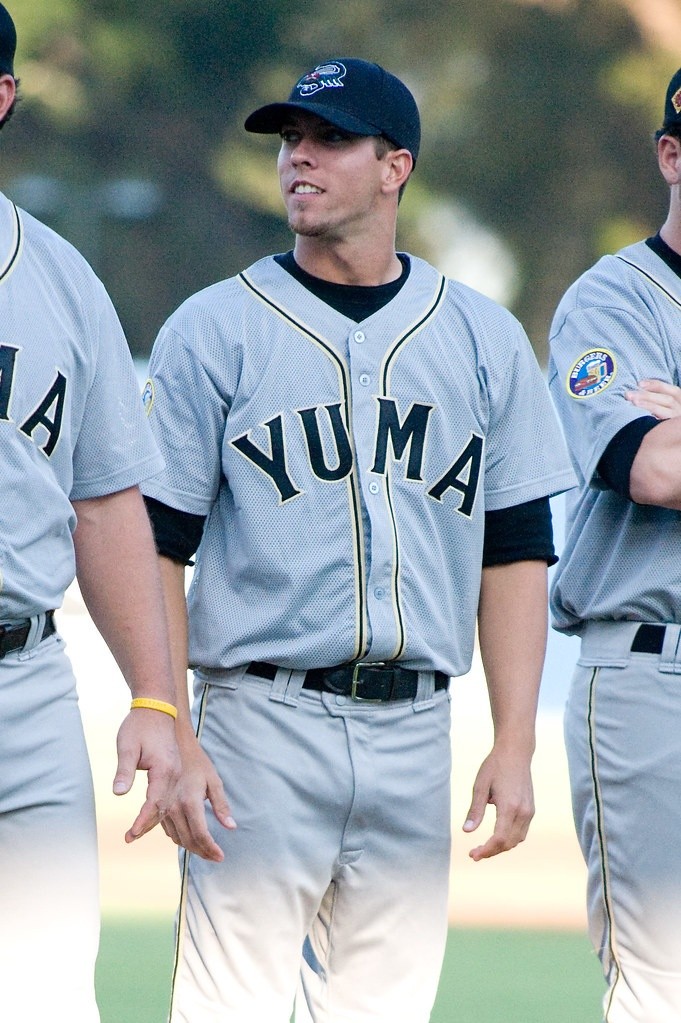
left=128, top=697, right=178, bottom=721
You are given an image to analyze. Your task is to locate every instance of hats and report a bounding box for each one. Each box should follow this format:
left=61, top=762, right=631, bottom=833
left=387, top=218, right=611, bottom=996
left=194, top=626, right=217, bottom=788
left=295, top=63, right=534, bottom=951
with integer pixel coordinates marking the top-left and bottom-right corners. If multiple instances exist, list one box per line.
left=663, top=68, right=681, bottom=137
left=0, top=4, right=17, bottom=78
left=245, top=57, right=421, bottom=171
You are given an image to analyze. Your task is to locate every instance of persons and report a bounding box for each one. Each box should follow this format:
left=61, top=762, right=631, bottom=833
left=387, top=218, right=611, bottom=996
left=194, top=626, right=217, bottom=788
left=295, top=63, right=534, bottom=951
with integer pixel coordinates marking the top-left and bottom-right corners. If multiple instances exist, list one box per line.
left=1, top=5, right=183, bottom=1022
left=548, top=64, right=681, bottom=1023
left=141, top=54, right=582, bottom=1023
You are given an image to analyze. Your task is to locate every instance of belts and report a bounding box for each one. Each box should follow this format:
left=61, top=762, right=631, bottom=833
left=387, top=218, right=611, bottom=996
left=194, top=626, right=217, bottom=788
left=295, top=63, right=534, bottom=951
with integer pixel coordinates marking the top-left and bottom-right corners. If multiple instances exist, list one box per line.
left=631, top=624, right=666, bottom=655
left=245, top=661, right=449, bottom=705
left=0, top=609, right=55, bottom=659
